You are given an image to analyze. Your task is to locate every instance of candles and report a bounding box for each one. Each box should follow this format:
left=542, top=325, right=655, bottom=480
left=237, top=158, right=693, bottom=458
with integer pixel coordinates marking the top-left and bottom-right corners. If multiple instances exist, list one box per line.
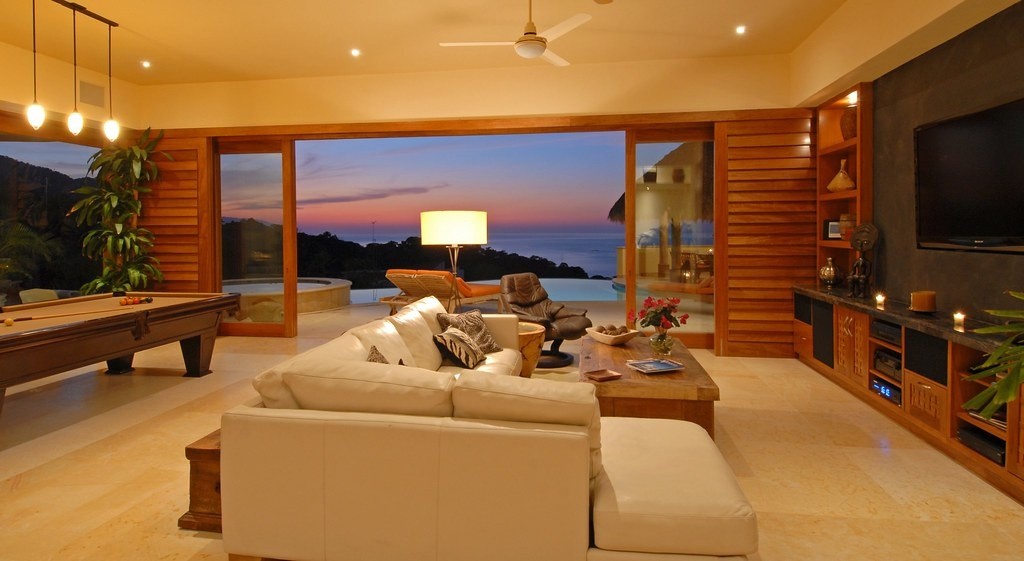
left=910, top=291, right=937, bottom=311
left=876, top=294, right=885, bottom=305
left=953, top=312, right=965, bottom=326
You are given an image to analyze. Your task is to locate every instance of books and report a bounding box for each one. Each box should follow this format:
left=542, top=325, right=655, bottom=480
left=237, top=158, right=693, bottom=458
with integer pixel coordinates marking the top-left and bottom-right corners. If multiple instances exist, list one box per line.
left=626, top=358, right=685, bottom=373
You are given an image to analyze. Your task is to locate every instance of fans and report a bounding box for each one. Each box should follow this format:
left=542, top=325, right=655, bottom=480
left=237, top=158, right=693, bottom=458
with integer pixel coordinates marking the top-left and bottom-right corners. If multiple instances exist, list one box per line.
left=439, top=0, right=593, bottom=68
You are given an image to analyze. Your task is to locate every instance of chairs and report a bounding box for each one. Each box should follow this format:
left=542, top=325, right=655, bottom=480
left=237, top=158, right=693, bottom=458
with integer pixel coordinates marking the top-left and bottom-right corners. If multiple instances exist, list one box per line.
left=499, top=272, right=592, bottom=368
left=680, top=251, right=711, bottom=283
left=378, top=268, right=501, bottom=316
left=697, top=252, right=715, bottom=276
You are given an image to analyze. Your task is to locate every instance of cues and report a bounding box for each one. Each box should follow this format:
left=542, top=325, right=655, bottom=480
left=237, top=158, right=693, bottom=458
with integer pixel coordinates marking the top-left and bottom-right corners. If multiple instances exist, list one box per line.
left=0, top=307, right=134, bottom=323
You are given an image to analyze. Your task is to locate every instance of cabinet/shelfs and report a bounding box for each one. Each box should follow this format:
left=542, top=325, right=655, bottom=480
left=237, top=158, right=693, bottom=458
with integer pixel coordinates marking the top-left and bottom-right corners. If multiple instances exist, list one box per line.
left=636, top=162, right=693, bottom=276
left=792, top=291, right=1024, bottom=507
left=816, top=80, right=873, bottom=284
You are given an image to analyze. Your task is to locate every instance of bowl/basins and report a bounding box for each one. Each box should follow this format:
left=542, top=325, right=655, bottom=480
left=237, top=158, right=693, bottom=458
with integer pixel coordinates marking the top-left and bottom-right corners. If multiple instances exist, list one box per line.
left=585, top=327, right=641, bottom=346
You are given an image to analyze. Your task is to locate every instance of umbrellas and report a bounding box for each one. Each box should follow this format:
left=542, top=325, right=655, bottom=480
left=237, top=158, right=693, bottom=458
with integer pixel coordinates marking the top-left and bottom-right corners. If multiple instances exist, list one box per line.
left=607, top=142, right=715, bottom=223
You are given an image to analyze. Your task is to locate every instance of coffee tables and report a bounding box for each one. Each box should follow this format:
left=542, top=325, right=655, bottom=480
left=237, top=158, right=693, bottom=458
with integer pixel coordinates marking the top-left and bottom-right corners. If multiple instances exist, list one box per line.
left=578, top=335, right=719, bottom=441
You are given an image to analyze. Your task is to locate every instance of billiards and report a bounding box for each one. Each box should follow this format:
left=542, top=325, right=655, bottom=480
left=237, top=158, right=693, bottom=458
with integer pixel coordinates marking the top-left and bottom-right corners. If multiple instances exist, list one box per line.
left=5, top=318, right=14, bottom=326
left=119, top=296, right=154, bottom=306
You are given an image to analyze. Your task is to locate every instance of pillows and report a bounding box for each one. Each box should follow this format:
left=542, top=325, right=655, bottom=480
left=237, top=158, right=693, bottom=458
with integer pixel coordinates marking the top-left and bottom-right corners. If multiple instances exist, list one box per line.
left=432, top=325, right=488, bottom=369
left=366, top=345, right=390, bottom=365
left=436, top=309, right=503, bottom=354
left=456, top=277, right=472, bottom=292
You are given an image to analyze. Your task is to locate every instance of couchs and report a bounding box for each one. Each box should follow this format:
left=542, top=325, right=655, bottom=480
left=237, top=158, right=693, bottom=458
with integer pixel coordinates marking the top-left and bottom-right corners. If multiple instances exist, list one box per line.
left=0, top=289, right=59, bottom=308
left=220, top=296, right=758, bottom=561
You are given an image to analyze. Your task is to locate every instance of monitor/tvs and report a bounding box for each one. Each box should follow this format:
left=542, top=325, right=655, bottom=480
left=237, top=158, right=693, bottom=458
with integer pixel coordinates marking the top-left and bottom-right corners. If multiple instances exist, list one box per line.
left=913, top=97, right=1024, bottom=254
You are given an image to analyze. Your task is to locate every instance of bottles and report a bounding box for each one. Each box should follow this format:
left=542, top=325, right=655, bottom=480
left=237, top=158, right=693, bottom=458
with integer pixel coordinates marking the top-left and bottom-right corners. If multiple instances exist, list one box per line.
left=819, top=258, right=845, bottom=287
left=838, top=214, right=853, bottom=240
left=840, top=108, right=857, bottom=141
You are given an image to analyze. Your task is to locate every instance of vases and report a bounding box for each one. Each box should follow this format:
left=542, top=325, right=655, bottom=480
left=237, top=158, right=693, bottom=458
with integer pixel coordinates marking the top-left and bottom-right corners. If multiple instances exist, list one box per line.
left=672, top=169, right=685, bottom=184
left=818, top=256, right=843, bottom=284
left=648, top=325, right=674, bottom=356
left=839, top=105, right=857, bottom=141
left=827, top=160, right=855, bottom=193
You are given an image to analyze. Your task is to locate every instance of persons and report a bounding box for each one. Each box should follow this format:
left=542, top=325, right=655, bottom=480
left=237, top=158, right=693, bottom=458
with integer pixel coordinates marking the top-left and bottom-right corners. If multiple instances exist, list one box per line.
left=846, top=251, right=870, bottom=298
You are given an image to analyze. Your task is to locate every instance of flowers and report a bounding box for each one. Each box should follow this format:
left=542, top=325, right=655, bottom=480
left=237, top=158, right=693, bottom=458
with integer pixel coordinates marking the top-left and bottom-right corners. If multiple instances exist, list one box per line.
left=626, top=296, right=690, bottom=343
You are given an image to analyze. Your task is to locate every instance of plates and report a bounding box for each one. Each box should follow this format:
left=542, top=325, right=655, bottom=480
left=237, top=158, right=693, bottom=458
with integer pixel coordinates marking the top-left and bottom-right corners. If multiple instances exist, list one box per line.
left=905, top=308, right=944, bottom=314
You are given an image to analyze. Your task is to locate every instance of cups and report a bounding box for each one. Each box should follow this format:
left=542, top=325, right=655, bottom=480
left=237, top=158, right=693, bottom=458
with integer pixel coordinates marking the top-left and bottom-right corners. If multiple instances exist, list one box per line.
left=642, top=166, right=657, bottom=183
left=672, top=168, right=684, bottom=183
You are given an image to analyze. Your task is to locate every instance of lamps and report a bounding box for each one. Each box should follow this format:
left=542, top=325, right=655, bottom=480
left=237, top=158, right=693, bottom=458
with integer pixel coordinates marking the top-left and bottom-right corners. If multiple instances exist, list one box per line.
left=26, top=0, right=45, bottom=129
left=68, top=8, right=84, bottom=135
left=420, top=210, right=487, bottom=314
left=103, top=24, right=120, bottom=141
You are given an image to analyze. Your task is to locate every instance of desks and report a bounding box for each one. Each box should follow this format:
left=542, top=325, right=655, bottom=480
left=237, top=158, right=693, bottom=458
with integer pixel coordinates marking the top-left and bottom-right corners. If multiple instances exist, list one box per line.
left=0, top=288, right=242, bottom=406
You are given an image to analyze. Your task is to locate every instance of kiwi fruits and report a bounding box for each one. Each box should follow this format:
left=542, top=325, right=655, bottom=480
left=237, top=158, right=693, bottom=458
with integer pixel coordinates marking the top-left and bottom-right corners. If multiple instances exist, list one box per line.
left=596, top=324, right=627, bottom=336
